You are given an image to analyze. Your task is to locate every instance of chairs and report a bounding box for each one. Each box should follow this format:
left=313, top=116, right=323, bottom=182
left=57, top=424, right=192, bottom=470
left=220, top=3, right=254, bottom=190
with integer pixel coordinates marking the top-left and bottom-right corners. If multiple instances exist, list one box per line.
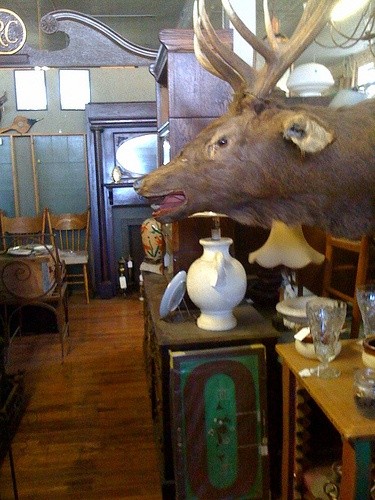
left=0, top=209, right=89, bottom=363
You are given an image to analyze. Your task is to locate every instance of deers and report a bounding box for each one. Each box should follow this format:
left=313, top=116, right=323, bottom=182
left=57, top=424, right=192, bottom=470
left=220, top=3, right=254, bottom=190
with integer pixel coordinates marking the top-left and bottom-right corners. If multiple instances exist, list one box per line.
left=132, top=0, right=375, bottom=241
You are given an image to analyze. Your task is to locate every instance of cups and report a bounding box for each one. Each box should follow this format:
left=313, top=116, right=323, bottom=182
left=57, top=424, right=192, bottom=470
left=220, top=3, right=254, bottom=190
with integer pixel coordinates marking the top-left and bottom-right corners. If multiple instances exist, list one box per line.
left=361, top=337, right=375, bottom=369
left=353, top=367, right=375, bottom=410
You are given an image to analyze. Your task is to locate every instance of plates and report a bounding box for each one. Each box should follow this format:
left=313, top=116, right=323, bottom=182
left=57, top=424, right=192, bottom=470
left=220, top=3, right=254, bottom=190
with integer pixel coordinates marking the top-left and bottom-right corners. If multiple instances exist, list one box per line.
left=6, top=244, right=55, bottom=257
left=160, top=271, right=186, bottom=319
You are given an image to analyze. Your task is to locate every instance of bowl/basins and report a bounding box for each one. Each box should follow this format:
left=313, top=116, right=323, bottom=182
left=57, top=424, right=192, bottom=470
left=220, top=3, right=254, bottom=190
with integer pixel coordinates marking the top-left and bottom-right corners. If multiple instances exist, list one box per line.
left=295, top=334, right=343, bottom=360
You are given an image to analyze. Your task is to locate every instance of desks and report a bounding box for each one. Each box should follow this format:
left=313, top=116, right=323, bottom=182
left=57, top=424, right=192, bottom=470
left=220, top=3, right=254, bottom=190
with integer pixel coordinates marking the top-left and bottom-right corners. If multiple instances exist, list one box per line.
left=0, top=249, right=89, bottom=270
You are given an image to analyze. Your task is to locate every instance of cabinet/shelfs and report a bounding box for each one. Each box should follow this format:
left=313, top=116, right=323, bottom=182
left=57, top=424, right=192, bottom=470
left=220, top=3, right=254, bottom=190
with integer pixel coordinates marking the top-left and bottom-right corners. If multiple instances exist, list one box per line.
left=154, top=26, right=234, bottom=171
left=272, top=339, right=375, bottom=500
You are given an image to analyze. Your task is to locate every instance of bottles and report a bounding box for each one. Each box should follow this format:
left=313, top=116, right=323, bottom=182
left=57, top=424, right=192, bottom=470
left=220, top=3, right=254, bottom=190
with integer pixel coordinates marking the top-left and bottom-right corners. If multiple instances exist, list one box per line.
left=186, top=236, right=246, bottom=330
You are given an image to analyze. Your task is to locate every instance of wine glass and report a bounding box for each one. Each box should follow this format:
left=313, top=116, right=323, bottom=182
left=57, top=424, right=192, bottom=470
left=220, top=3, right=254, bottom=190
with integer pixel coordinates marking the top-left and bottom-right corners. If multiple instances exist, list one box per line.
left=354, top=284, right=375, bottom=352
left=306, top=298, right=347, bottom=377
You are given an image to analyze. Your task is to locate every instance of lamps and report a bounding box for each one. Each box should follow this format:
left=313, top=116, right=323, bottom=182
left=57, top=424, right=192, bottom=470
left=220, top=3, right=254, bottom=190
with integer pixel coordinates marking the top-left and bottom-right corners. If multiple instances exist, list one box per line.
left=283, top=60, right=333, bottom=99
left=183, top=210, right=249, bottom=331
left=249, top=218, right=326, bottom=331
